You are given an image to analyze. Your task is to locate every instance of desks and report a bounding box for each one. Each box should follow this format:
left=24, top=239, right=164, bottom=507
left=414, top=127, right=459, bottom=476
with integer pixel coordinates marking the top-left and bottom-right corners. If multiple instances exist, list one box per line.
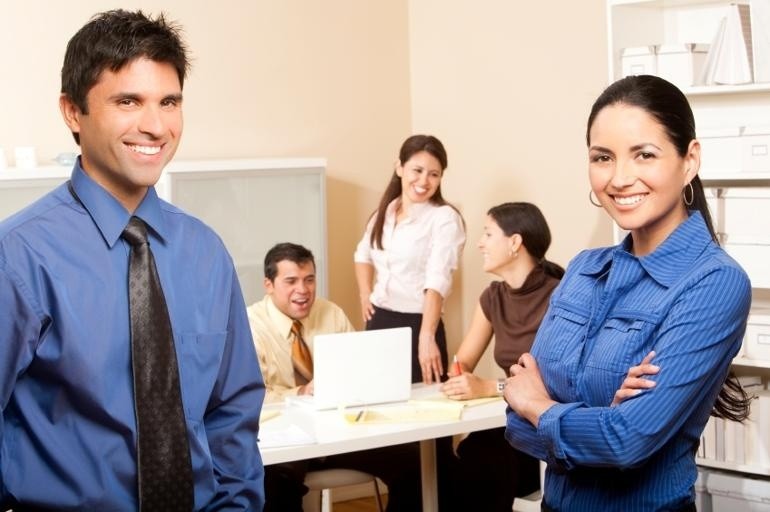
left=243, top=379, right=511, bottom=512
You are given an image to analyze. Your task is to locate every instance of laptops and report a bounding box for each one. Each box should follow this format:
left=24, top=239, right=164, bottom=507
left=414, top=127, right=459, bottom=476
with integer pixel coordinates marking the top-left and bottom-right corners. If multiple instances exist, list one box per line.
left=284, top=326, right=413, bottom=413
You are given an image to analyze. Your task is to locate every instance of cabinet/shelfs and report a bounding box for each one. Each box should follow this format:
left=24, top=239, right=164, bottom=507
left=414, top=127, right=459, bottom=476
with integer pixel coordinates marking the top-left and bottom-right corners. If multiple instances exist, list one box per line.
left=602, top=2, right=770, bottom=200
left=684, top=284, right=770, bottom=479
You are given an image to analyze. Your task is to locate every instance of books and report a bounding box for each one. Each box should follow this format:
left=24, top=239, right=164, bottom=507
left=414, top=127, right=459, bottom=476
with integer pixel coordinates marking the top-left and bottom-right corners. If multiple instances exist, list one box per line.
left=695, top=287, right=770, bottom=472
left=696, top=4, right=753, bottom=85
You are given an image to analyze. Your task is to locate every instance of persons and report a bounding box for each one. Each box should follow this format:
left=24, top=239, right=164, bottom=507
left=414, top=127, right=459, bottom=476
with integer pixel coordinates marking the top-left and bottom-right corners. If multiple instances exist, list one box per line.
left=245, top=242, right=356, bottom=512
left=355, top=135, right=466, bottom=459
left=446, top=203, right=566, bottom=512
left=505, top=75, right=752, bottom=512
left=0, top=8, right=264, bottom=511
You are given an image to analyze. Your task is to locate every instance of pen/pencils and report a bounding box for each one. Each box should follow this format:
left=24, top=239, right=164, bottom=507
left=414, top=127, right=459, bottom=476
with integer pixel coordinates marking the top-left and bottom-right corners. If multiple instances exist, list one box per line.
left=355, top=410, right=364, bottom=422
left=453, top=354, right=462, bottom=377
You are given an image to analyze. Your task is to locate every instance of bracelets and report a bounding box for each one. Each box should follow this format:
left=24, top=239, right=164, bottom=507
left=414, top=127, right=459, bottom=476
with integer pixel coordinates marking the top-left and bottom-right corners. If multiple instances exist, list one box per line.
left=496, top=378, right=504, bottom=395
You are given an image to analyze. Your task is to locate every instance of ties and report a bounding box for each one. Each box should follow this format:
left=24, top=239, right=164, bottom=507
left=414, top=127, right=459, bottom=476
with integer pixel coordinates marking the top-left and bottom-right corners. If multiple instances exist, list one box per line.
left=291, top=321, right=313, bottom=384
left=122, top=217, right=194, bottom=512
